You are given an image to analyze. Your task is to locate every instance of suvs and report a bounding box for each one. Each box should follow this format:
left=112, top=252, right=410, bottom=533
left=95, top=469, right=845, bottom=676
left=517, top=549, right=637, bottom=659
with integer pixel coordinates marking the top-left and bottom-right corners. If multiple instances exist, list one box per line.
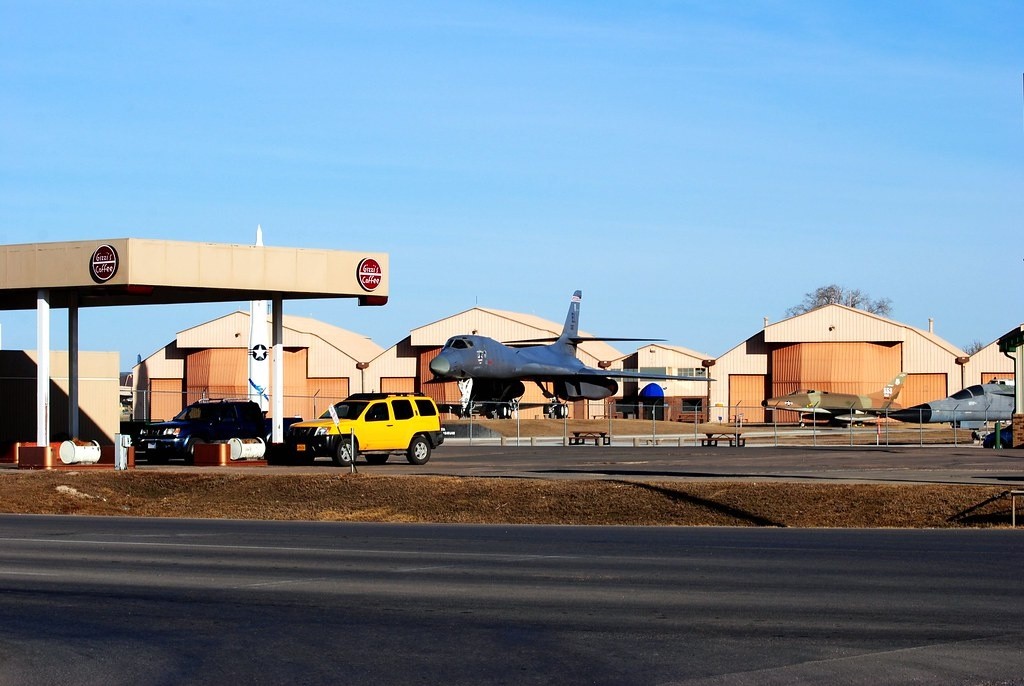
left=290, top=392, right=446, bottom=467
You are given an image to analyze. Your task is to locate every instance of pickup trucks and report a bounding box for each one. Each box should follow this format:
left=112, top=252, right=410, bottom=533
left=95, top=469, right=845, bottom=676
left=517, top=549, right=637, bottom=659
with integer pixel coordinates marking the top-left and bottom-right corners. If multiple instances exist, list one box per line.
left=139, top=397, right=303, bottom=470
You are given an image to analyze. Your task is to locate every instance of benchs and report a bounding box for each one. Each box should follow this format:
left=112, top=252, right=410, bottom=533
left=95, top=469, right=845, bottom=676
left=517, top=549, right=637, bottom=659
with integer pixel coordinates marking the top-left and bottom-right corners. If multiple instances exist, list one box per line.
left=569, top=437, right=610, bottom=445
left=699, top=438, right=747, bottom=447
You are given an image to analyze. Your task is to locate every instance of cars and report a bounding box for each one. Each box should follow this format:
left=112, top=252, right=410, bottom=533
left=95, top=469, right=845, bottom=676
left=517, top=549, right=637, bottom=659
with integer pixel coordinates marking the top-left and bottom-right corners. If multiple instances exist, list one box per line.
left=983, top=424, right=1013, bottom=450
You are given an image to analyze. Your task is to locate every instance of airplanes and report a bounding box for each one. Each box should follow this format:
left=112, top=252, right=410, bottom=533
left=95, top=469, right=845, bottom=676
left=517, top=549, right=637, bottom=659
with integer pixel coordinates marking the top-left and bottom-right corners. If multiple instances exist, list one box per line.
left=422, top=289, right=717, bottom=419
left=886, top=379, right=1015, bottom=440
left=760, top=371, right=908, bottom=427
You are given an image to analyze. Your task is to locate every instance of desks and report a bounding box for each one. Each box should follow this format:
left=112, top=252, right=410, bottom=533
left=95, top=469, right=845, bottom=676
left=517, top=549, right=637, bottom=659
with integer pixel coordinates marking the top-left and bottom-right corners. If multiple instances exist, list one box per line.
left=570, top=431, right=607, bottom=437
left=703, top=432, right=742, bottom=438
